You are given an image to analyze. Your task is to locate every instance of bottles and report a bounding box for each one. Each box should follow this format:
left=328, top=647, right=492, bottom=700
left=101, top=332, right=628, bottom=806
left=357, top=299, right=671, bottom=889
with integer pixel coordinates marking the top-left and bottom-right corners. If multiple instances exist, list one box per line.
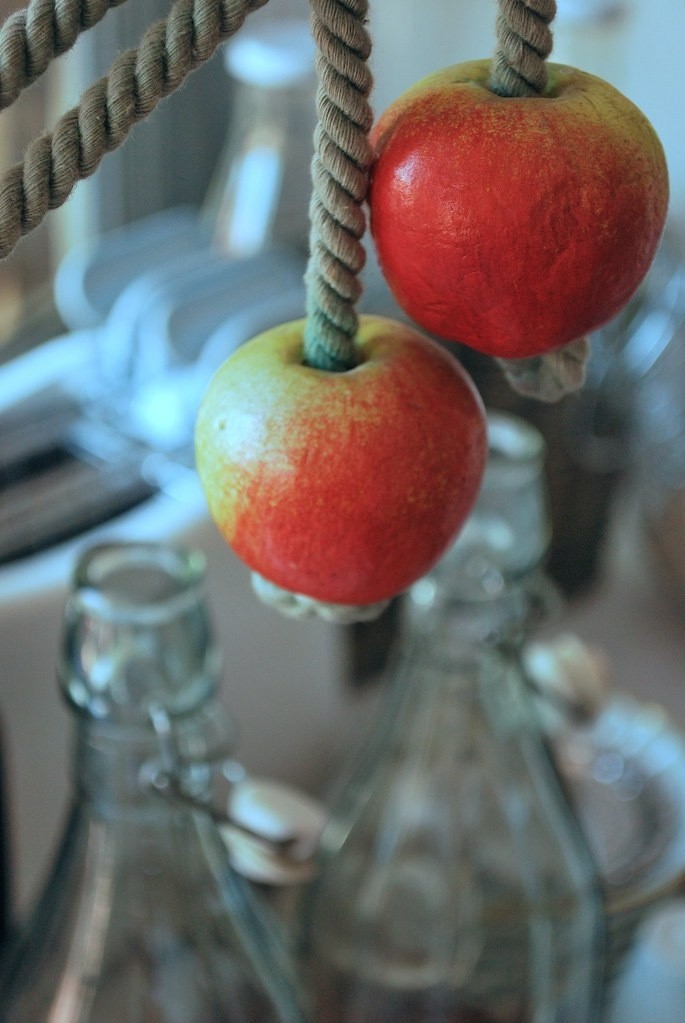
left=2, top=539, right=311, bottom=1022
left=292, top=409, right=615, bottom=1023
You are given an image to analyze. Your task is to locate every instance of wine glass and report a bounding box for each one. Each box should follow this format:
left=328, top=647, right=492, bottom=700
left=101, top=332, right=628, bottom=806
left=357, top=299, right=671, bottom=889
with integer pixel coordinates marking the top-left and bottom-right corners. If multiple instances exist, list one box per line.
left=451, top=191, right=683, bottom=926
